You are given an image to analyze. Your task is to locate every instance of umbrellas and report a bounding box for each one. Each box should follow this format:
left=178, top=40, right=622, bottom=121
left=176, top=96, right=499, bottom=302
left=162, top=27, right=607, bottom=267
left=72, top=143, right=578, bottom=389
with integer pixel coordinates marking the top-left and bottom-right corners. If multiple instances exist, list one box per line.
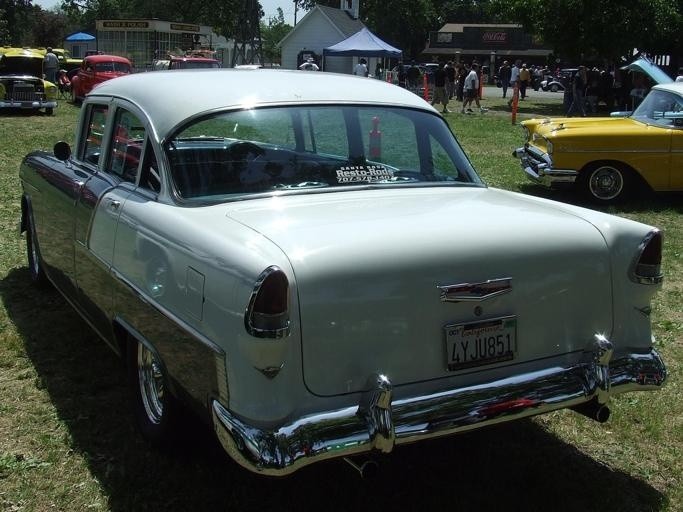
left=67, top=32, right=95, bottom=59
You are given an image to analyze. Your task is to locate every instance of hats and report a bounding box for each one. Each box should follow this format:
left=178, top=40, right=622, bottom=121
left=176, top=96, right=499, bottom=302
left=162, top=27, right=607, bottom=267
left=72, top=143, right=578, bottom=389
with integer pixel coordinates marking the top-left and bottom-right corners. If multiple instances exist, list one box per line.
left=60, top=69, right=68, bottom=73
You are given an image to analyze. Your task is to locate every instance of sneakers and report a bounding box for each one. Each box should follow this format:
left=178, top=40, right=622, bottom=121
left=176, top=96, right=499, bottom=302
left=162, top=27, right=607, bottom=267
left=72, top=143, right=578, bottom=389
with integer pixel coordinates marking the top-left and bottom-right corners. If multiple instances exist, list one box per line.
left=443, top=108, right=489, bottom=114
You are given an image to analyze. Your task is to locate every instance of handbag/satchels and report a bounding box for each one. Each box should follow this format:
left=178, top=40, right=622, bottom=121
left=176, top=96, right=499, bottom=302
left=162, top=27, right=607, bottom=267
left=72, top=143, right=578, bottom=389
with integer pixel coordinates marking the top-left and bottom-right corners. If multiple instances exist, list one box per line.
left=497, top=80, right=503, bottom=88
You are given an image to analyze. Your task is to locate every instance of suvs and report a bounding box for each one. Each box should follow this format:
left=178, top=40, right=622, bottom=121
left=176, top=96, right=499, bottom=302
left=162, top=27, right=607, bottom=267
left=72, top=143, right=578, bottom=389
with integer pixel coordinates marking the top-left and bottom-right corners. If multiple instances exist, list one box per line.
left=539, top=69, right=579, bottom=93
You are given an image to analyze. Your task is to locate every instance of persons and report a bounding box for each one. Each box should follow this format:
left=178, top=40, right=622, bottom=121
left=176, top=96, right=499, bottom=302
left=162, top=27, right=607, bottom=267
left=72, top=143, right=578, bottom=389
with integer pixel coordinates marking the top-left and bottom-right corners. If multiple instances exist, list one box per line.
left=43, top=47, right=59, bottom=84
left=299, top=57, right=320, bottom=71
left=58, top=70, right=72, bottom=93
left=675, top=67, right=683, bottom=83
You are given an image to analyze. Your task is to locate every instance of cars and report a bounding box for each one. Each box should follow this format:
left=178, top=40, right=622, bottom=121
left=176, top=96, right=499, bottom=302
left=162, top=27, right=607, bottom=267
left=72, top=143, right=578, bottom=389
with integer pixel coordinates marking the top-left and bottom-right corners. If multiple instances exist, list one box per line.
left=609, top=57, right=683, bottom=119
left=391, top=63, right=470, bottom=86
left=17, top=67, right=666, bottom=481
left=511, top=81, right=683, bottom=206
left=0, top=45, right=263, bottom=115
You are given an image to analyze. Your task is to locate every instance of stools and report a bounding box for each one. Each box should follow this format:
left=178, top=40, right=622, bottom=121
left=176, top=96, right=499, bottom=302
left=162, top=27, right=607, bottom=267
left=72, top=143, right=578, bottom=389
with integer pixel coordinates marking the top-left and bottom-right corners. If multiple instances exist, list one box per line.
left=56, top=86, right=73, bottom=100
